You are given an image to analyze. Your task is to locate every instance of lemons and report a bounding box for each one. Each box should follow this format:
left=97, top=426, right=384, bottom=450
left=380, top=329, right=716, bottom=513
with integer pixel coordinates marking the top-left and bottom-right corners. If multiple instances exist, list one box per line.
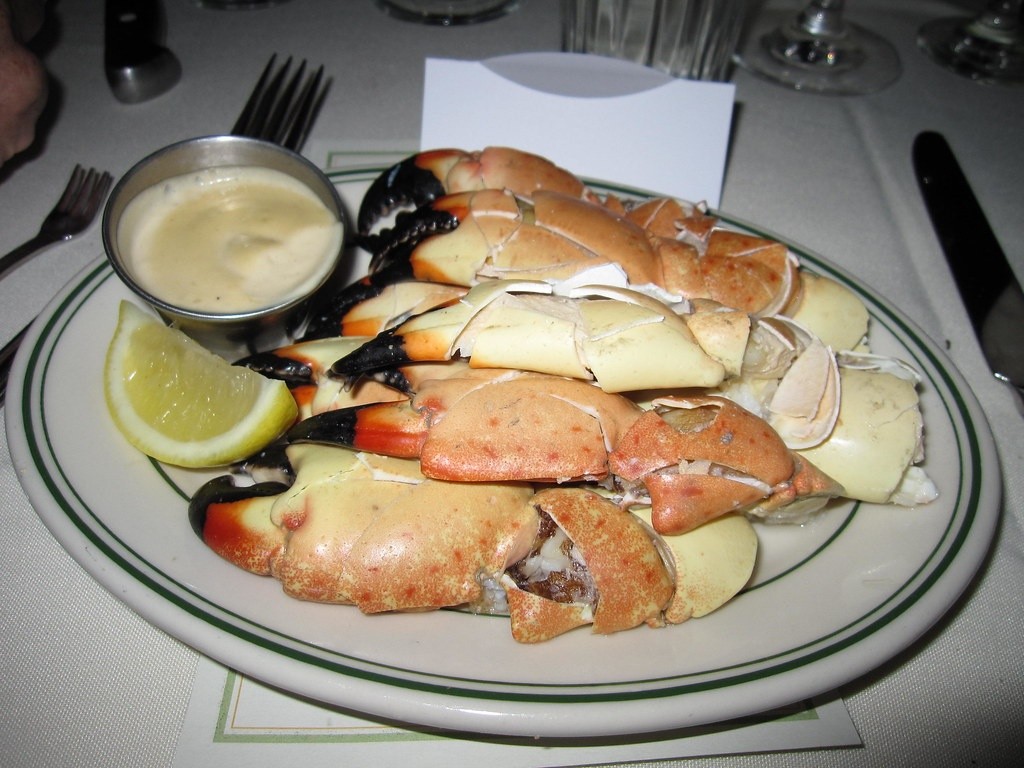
left=104, top=297, right=299, bottom=468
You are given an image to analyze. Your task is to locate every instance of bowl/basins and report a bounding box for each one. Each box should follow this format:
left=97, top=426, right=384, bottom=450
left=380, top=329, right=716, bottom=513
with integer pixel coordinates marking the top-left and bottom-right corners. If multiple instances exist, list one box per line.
left=101, top=134, right=348, bottom=334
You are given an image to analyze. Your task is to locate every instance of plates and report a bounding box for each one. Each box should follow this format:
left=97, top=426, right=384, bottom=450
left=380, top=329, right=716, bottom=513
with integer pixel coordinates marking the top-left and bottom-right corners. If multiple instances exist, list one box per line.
left=5, top=163, right=1003, bottom=738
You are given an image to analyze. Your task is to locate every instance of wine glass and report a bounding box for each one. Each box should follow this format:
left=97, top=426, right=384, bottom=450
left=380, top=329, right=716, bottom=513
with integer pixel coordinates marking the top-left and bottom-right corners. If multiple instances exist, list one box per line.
left=913, top=0, right=1024, bottom=89
left=731, top=0, right=903, bottom=96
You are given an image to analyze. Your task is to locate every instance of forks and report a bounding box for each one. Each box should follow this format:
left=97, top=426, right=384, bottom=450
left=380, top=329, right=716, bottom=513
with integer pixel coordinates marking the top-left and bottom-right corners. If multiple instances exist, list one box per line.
left=0, top=164, right=110, bottom=278
left=0, top=51, right=324, bottom=406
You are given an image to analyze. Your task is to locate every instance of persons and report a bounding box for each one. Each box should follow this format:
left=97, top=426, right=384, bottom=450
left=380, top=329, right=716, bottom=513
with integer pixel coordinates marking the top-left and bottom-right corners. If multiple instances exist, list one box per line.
left=0, top=0, right=49, bottom=161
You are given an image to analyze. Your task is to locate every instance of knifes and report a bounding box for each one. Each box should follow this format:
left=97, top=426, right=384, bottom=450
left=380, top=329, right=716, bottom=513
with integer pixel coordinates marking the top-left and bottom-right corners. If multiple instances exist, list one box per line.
left=912, top=130, right=1024, bottom=402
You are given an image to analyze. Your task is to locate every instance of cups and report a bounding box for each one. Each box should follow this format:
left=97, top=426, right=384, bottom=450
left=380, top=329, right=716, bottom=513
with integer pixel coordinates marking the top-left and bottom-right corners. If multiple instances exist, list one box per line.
left=559, top=0, right=752, bottom=83
